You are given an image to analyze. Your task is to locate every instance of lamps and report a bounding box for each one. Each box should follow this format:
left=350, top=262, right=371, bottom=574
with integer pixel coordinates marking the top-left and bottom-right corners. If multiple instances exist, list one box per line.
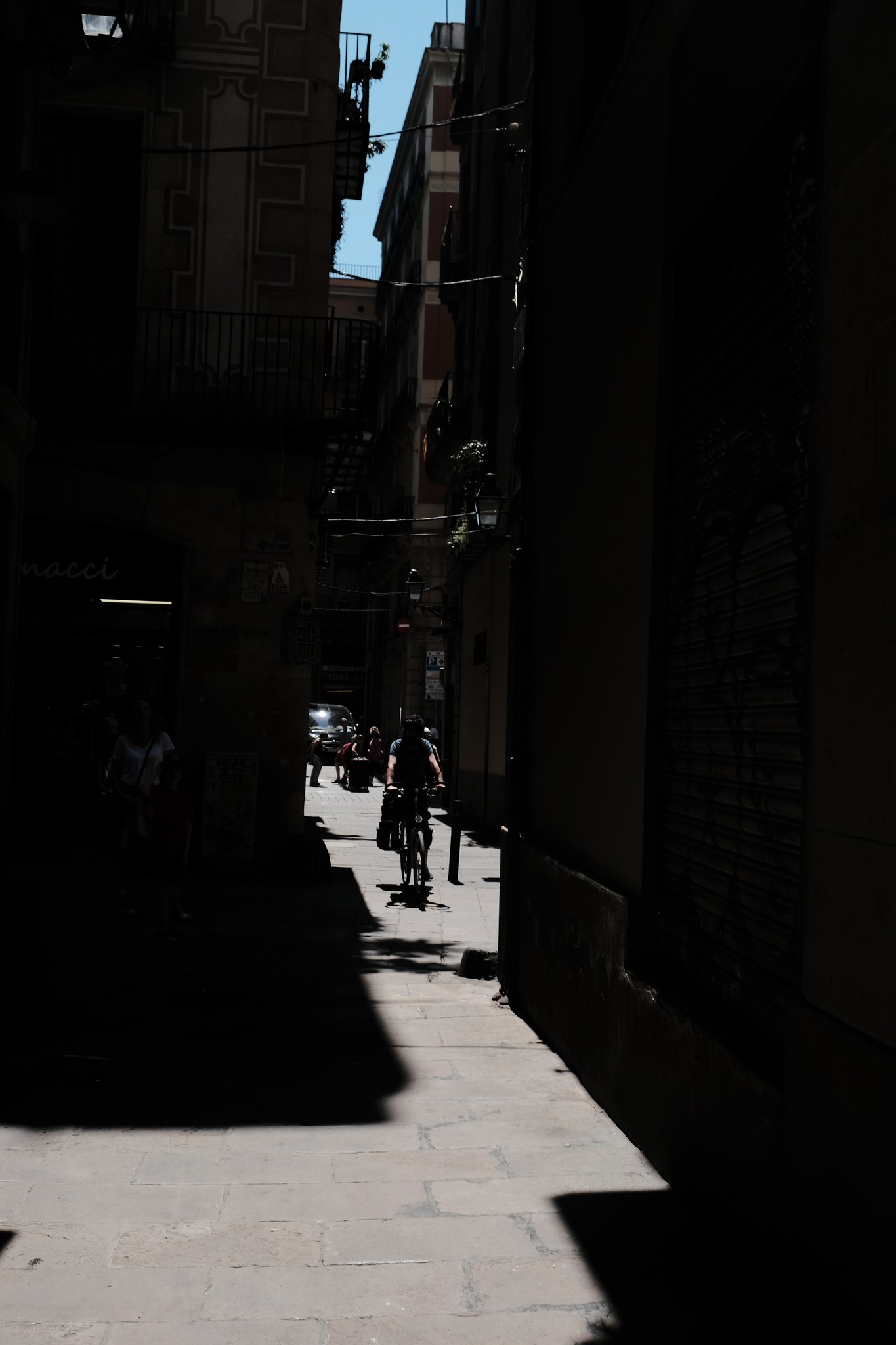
left=472, top=472, right=511, bottom=535
left=407, top=570, right=448, bottom=621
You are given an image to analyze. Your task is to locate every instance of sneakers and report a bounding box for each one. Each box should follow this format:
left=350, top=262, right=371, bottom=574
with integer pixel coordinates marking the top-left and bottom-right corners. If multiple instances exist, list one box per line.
left=426, top=865, right=434, bottom=881
left=390, top=833, right=401, bottom=851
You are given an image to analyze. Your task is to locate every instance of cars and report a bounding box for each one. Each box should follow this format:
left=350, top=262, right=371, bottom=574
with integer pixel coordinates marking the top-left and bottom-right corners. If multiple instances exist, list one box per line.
left=309, top=704, right=360, bottom=753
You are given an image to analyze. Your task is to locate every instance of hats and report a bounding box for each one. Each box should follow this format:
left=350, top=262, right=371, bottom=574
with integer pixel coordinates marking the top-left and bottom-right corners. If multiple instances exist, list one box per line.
left=424, top=727, right=430, bottom=734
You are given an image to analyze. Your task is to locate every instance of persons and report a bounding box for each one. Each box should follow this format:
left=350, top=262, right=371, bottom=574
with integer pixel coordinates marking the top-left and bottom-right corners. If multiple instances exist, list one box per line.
left=387, top=715, right=444, bottom=882
left=109, top=693, right=195, bottom=926
left=311, top=715, right=438, bottom=787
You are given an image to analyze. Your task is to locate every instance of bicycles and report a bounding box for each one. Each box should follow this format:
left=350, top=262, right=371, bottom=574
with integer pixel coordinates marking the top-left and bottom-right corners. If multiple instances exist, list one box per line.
left=377, top=779, right=451, bottom=901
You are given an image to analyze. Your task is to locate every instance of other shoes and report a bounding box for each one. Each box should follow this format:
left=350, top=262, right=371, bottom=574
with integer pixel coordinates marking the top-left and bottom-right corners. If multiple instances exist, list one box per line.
left=331, top=779, right=341, bottom=784
